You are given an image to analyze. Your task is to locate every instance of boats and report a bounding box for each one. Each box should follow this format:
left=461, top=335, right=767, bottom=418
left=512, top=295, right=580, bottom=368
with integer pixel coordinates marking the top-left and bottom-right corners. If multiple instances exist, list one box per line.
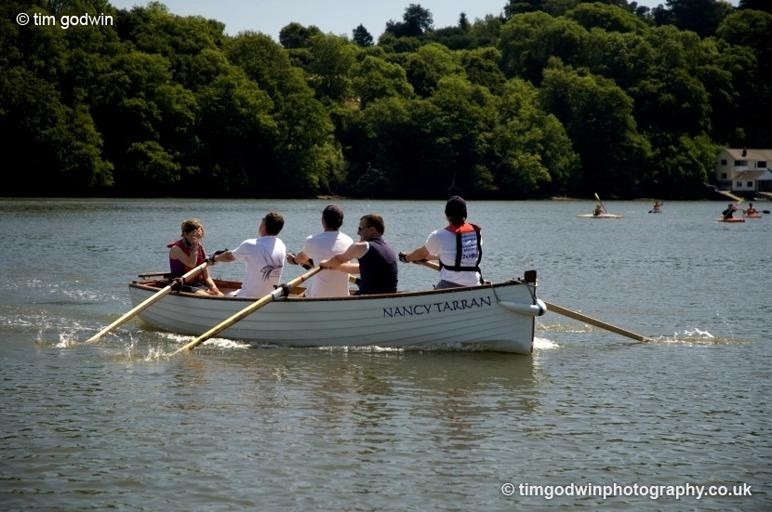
left=130, top=269, right=547, bottom=355
left=716, top=212, right=762, bottom=222
left=577, top=213, right=624, bottom=219
left=648, top=208, right=662, bottom=213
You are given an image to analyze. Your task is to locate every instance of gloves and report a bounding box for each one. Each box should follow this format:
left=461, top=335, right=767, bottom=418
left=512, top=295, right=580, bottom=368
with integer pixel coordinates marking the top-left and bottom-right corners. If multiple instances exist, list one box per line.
left=398, top=252, right=409, bottom=263
left=412, top=257, right=429, bottom=264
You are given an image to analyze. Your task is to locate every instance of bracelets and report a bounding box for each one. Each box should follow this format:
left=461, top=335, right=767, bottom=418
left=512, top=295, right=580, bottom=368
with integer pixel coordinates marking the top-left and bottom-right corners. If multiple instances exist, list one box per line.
left=292, top=258, right=298, bottom=265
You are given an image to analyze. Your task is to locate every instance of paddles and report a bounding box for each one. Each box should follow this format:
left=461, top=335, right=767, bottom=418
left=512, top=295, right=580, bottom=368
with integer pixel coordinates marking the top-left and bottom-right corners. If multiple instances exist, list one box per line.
left=723, top=197, right=743, bottom=218
left=754, top=210, right=770, bottom=214
left=648, top=202, right=663, bottom=213
left=595, top=193, right=608, bottom=213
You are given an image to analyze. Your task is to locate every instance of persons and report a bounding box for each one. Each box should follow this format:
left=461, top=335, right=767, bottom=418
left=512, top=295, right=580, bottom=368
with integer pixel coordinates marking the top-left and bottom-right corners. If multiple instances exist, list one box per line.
left=400, top=196, right=483, bottom=290
left=743, top=203, right=759, bottom=215
left=169, top=218, right=225, bottom=297
left=653, top=199, right=663, bottom=210
left=204, top=213, right=286, bottom=298
left=593, top=204, right=606, bottom=215
left=287, top=204, right=354, bottom=297
left=722, top=203, right=737, bottom=219
left=319, top=215, right=397, bottom=294
